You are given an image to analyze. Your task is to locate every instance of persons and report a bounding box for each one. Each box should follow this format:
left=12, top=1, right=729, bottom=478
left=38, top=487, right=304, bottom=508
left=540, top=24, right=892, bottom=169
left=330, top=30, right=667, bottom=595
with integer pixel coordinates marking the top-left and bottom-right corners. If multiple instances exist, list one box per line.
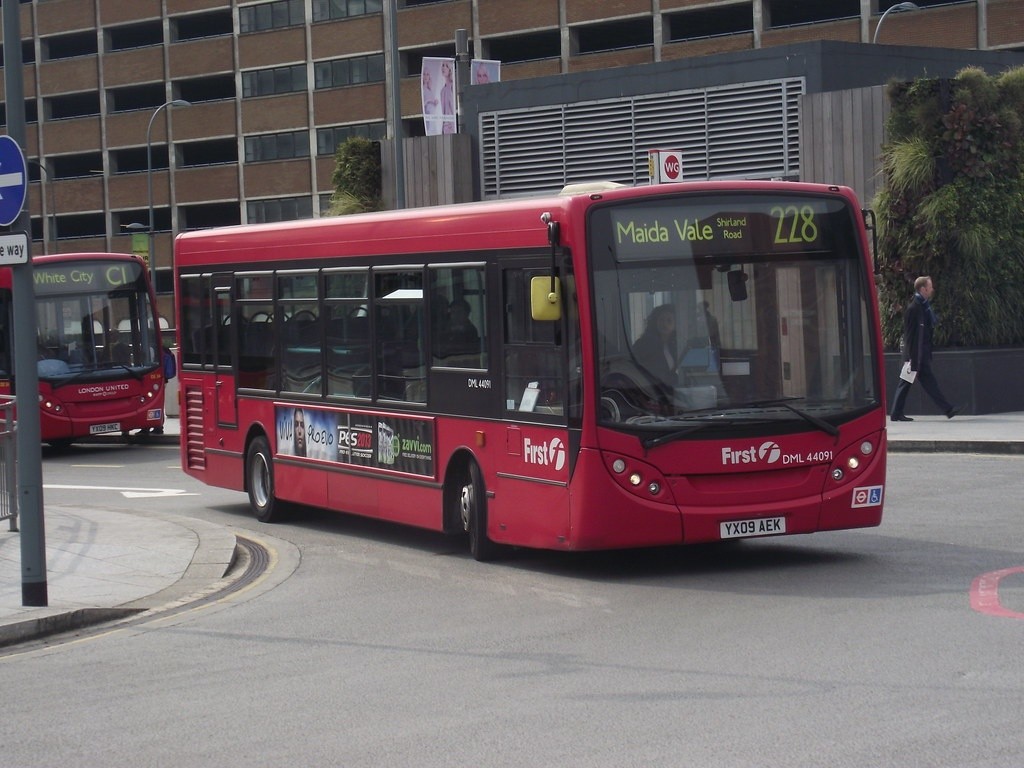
left=890, top=276, right=968, bottom=421
left=135, top=329, right=165, bottom=434
left=448, top=299, right=478, bottom=349
left=293, top=408, right=307, bottom=457
left=697, top=301, right=721, bottom=346
left=476, top=62, right=489, bottom=84
left=634, top=303, right=677, bottom=366
left=441, top=61, right=456, bottom=134
left=47, top=329, right=58, bottom=347
left=423, top=67, right=439, bottom=112
left=111, top=329, right=130, bottom=364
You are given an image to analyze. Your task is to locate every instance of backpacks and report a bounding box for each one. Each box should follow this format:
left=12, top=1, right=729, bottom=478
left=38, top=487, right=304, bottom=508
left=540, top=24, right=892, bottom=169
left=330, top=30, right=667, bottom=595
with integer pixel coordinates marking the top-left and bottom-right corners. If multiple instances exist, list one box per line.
left=151, top=344, right=176, bottom=379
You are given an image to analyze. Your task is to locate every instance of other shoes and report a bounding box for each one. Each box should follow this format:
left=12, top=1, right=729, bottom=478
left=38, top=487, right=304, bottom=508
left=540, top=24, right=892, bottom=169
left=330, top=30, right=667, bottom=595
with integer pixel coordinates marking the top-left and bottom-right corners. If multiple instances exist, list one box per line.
left=891, top=416, right=913, bottom=421
left=151, top=429, right=163, bottom=434
left=947, top=403, right=966, bottom=418
left=136, top=430, right=149, bottom=434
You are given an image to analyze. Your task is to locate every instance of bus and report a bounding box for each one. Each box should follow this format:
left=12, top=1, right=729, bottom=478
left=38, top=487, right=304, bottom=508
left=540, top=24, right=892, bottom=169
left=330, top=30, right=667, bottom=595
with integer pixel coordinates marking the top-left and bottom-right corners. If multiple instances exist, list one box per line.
left=172, top=179, right=889, bottom=563
left=0, top=250, right=166, bottom=447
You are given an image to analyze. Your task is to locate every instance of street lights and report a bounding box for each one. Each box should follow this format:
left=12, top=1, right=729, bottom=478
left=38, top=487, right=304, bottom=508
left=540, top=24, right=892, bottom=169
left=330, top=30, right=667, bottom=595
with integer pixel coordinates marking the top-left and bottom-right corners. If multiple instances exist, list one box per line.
left=128, top=97, right=195, bottom=359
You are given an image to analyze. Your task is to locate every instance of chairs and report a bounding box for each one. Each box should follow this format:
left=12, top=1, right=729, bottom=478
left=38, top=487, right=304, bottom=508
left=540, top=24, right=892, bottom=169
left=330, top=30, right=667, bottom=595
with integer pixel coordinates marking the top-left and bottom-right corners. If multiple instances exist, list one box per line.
left=197, top=299, right=447, bottom=364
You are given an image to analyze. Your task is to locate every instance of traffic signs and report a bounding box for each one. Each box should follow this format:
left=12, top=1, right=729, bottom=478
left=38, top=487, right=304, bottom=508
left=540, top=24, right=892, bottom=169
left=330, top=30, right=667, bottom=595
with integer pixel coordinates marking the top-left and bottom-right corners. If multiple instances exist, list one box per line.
left=0, top=229, right=31, bottom=266
